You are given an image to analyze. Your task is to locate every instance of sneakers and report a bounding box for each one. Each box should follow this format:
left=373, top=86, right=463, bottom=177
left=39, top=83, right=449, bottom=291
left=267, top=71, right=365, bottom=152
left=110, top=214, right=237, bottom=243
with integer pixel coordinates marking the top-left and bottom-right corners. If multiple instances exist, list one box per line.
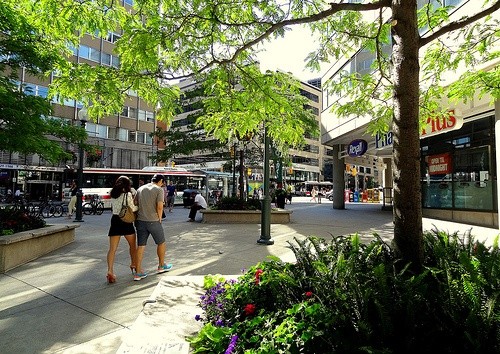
left=157, top=264, right=173, bottom=273
left=133, top=272, right=147, bottom=281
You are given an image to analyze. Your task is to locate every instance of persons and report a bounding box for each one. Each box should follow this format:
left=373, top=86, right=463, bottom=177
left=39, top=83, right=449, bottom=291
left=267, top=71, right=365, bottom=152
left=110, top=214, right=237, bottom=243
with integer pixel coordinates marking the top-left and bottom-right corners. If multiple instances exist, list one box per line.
left=134, top=173, right=172, bottom=280
left=317, top=185, right=322, bottom=204
left=188, top=192, right=207, bottom=222
left=67, top=179, right=79, bottom=218
left=167, top=180, right=177, bottom=213
left=106, top=176, right=139, bottom=283
left=310, top=186, right=317, bottom=202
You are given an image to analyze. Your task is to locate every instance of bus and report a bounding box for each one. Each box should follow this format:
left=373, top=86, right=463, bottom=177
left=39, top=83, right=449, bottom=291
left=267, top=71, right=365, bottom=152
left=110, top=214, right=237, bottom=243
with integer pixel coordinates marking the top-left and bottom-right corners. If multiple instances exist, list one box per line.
left=191, top=169, right=240, bottom=202
left=61, top=165, right=207, bottom=209
left=303, top=181, right=333, bottom=197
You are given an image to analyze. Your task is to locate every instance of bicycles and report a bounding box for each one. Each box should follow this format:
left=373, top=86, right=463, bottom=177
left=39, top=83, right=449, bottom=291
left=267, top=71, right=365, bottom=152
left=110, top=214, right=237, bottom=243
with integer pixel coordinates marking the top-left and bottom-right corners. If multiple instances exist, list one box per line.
left=82, top=194, right=105, bottom=216
left=0, top=193, right=64, bottom=219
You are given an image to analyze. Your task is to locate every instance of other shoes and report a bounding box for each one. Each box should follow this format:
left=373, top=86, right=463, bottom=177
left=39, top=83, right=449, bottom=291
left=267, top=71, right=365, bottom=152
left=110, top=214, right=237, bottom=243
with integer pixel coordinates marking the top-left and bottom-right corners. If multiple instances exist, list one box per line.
left=162, top=217, right=167, bottom=221
left=187, top=219, right=195, bottom=222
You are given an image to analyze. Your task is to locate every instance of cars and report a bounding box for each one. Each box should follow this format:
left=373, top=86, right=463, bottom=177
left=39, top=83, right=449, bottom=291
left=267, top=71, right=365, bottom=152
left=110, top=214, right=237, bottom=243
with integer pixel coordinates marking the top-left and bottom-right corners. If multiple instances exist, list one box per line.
left=325, top=189, right=349, bottom=201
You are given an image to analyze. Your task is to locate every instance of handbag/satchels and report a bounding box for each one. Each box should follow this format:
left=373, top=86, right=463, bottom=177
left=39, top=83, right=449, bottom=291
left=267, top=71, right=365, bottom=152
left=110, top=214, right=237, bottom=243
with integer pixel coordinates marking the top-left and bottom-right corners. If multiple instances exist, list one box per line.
left=119, top=192, right=135, bottom=224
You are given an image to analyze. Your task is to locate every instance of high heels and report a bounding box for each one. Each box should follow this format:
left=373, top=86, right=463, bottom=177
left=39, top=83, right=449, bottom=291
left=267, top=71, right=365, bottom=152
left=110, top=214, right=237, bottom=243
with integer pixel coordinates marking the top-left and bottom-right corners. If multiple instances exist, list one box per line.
left=106, top=273, right=115, bottom=285
left=129, top=264, right=136, bottom=273
